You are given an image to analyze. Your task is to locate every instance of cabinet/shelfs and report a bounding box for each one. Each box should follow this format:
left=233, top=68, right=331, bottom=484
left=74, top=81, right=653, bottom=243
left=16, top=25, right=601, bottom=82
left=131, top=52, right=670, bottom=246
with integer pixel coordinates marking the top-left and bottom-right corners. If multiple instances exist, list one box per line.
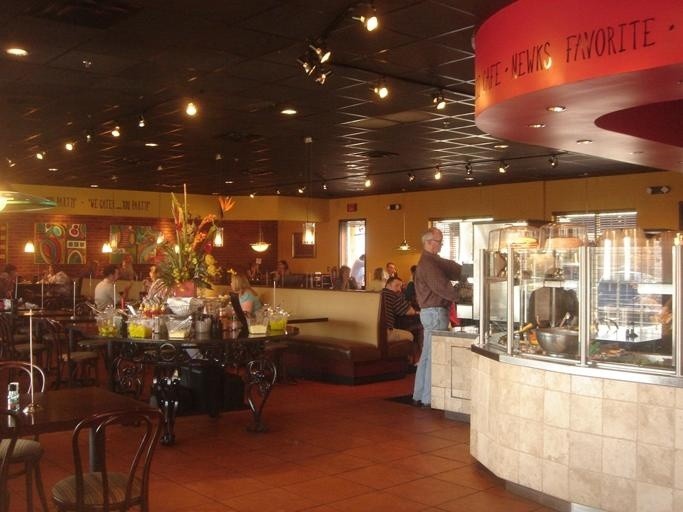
left=476, top=221, right=682, bottom=377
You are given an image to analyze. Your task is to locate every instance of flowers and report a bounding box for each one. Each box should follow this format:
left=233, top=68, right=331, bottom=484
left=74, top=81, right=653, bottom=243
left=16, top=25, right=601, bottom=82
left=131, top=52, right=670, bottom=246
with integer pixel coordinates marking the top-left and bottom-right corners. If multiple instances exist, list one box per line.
left=133, top=182, right=238, bottom=294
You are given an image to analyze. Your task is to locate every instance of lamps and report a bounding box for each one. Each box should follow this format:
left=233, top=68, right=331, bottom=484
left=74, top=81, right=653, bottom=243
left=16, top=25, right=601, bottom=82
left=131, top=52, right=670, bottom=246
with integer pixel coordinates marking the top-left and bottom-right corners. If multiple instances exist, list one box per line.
left=8, top=98, right=200, bottom=171
left=212, top=225, right=225, bottom=248
left=101, top=214, right=115, bottom=256
left=393, top=199, right=423, bottom=256
left=250, top=154, right=560, bottom=200
left=296, top=4, right=381, bottom=90
left=367, top=71, right=449, bottom=115
left=250, top=220, right=273, bottom=255
left=0, top=178, right=60, bottom=217
left=22, top=217, right=37, bottom=253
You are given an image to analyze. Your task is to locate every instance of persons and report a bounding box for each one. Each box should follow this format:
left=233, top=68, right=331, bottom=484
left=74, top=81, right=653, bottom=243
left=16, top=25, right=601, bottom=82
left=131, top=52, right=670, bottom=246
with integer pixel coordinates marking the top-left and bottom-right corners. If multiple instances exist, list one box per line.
left=239, top=250, right=421, bottom=307
left=379, top=275, right=414, bottom=346
left=228, top=272, right=265, bottom=315
left=36, top=261, right=71, bottom=306
left=93, top=264, right=132, bottom=309
left=0, top=262, right=17, bottom=300
left=527, top=268, right=582, bottom=333
left=409, top=226, right=473, bottom=408
left=140, top=264, right=168, bottom=307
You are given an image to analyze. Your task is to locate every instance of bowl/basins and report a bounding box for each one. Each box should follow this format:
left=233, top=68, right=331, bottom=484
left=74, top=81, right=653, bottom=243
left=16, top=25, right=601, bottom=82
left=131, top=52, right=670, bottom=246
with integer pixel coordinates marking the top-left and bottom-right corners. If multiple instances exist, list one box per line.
left=534, top=328, right=578, bottom=358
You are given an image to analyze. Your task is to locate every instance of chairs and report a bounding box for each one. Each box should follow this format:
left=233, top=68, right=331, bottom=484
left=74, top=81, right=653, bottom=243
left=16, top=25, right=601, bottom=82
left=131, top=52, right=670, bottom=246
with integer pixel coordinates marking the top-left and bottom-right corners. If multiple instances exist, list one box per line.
left=248, top=269, right=361, bottom=292
left=1, top=409, right=19, bottom=511
left=51, top=407, right=164, bottom=512
left=0, top=361, right=50, bottom=512
left=0, top=270, right=299, bottom=471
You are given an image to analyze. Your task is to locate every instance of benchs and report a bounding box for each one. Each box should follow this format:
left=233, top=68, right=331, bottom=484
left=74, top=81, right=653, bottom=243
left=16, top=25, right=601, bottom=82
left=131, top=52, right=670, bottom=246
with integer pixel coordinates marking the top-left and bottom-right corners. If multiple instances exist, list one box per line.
left=383, top=293, right=417, bottom=384
left=277, top=286, right=382, bottom=387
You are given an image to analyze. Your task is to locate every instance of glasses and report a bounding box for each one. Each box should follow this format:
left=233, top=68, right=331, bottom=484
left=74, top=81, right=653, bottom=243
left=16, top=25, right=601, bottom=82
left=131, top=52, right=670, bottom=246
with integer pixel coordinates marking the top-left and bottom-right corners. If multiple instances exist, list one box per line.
left=427, top=239, right=443, bottom=245
